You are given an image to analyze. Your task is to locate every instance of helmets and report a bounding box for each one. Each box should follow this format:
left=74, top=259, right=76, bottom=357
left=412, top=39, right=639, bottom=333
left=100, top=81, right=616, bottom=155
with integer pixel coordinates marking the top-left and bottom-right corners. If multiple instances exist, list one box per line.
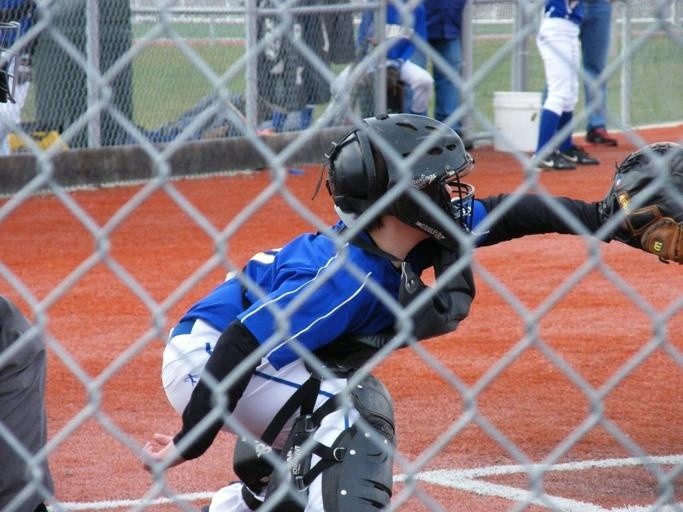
left=323, top=112, right=476, bottom=253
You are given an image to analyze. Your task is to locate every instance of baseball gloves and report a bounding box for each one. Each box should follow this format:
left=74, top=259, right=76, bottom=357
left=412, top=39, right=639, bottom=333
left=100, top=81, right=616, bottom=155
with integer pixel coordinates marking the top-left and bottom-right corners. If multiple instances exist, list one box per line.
left=598, top=142, right=683, bottom=263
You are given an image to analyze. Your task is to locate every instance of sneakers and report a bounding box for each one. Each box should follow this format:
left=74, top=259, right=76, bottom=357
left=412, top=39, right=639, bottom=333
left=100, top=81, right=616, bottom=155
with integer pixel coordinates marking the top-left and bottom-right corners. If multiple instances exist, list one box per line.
left=531, top=152, right=577, bottom=171
left=584, top=127, right=619, bottom=147
left=555, top=144, right=599, bottom=165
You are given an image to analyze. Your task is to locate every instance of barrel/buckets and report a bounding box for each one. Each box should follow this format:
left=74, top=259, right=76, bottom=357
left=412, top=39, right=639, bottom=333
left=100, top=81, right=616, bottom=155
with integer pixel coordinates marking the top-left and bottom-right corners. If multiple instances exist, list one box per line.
left=491, top=91, right=543, bottom=153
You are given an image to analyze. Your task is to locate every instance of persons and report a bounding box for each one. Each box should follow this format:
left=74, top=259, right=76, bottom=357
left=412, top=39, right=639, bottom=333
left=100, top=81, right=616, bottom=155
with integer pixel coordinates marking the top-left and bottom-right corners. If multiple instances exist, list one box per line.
left=579, top=0, right=618, bottom=147
left=136, top=113, right=683, bottom=512
left=327, top=1, right=435, bottom=117
left=532, top=1, right=599, bottom=171
left=0, top=0, right=41, bottom=157
left=401, top=0, right=475, bottom=151
